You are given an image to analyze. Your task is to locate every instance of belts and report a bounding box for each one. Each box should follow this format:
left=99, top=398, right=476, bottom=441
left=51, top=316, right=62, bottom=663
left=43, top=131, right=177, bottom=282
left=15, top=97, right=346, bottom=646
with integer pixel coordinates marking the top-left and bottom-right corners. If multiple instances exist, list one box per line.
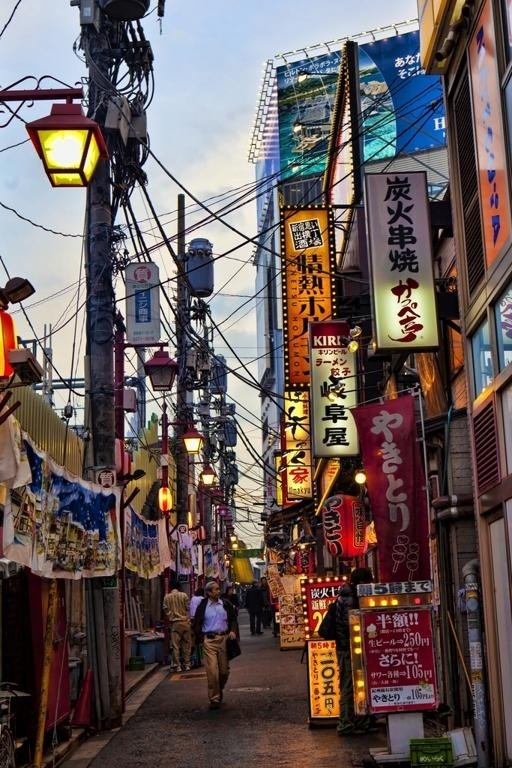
left=215, top=630, right=230, bottom=635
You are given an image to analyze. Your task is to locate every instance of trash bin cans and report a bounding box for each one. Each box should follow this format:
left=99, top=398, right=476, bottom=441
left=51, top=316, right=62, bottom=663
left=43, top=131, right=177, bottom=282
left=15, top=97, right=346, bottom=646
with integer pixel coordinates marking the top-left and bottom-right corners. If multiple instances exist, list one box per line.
left=131, top=630, right=165, bottom=664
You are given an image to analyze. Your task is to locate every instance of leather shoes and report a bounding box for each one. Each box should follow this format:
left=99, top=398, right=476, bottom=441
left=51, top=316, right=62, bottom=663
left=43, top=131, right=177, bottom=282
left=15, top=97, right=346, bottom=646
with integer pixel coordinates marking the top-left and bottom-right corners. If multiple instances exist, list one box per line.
left=209, top=690, right=223, bottom=710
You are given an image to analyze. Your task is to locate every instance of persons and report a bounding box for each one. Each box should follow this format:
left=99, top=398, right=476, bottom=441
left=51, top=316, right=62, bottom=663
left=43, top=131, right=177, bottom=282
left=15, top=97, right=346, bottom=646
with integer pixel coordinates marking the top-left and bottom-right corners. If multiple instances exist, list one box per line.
left=317, top=568, right=371, bottom=735
left=260, top=577, right=273, bottom=629
left=191, top=580, right=239, bottom=707
left=222, top=585, right=240, bottom=641
left=190, top=588, right=203, bottom=668
left=163, top=581, right=193, bottom=672
left=246, top=580, right=264, bottom=636
left=234, top=583, right=253, bottom=608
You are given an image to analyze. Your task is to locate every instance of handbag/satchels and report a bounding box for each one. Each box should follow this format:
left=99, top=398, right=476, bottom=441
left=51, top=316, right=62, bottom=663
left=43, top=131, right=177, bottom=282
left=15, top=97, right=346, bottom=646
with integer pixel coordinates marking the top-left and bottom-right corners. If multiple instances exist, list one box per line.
left=226, top=637, right=241, bottom=660
left=318, top=603, right=337, bottom=639
left=207, top=633, right=215, bottom=638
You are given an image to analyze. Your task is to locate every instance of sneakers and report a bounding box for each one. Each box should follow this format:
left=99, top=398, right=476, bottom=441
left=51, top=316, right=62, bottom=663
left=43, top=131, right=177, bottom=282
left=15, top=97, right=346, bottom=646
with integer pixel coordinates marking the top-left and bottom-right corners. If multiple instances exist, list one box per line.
left=174, top=666, right=190, bottom=672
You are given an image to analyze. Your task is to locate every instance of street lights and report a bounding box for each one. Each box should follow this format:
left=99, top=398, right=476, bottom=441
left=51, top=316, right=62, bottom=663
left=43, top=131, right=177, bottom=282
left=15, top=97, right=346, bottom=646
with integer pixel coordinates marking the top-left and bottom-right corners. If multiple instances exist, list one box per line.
left=0, top=89, right=110, bottom=186
